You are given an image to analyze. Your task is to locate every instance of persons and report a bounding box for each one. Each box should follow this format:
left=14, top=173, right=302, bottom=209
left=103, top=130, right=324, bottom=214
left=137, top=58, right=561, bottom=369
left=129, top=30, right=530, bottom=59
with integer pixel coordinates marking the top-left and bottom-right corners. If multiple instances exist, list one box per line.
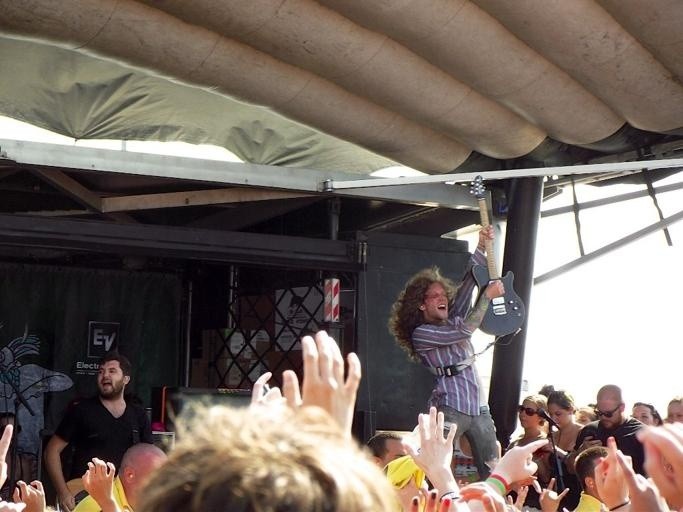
left=81, top=457, right=122, bottom=512
left=0, top=422, right=15, bottom=512
left=635, top=421, right=683, bottom=512
left=538, top=477, right=570, bottom=512
left=383, top=455, right=429, bottom=511
left=127, top=394, right=400, bottom=512
left=366, top=431, right=408, bottom=471
left=571, top=445, right=610, bottom=512
left=507, top=393, right=551, bottom=486
left=575, top=406, right=597, bottom=426
left=547, top=390, right=585, bottom=454
left=614, top=448, right=671, bottom=512
left=565, top=383, right=646, bottom=476
left=666, top=398, right=683, bottom=424
left=468, top=439, right=549, bottom=512
left=410, top=481, right=506, bottom=512
left=507, top=486, right=529, bottom=512
left=73, top=443, right=167, bottom=512
left=593, top=436, right=633, bottom=512
left=402, top=406, right=472, bottom=512
left=11, top=479, right=47, bottom=512
left=250, top=328, right=362, bottom=439
left=43, top=353, right=152, bottom=512
left=390, top=223, right=506, bottom=480
left=632, top=402, right=664, bottom=427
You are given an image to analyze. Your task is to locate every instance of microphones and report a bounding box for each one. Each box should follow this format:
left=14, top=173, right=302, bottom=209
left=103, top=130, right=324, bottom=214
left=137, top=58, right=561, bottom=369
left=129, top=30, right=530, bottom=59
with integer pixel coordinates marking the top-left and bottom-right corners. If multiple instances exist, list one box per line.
left=536, top=407, right=561, bottom=430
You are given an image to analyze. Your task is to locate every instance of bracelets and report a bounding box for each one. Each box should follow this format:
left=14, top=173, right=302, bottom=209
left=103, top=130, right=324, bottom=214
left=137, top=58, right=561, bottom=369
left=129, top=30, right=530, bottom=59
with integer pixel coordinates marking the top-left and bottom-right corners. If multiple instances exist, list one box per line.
left=609, top=501, right=630, bottom=511
left=485, top=474, right=510, bottom=495
left=439, top=491, right=460, bottom=501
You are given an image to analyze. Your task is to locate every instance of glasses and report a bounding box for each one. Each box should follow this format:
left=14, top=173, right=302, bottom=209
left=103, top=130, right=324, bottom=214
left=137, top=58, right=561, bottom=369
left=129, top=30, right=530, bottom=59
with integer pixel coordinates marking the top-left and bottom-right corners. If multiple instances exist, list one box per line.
left=594, top=404, right=619, bottom=418
left=518, top=405, right=538, bottom=416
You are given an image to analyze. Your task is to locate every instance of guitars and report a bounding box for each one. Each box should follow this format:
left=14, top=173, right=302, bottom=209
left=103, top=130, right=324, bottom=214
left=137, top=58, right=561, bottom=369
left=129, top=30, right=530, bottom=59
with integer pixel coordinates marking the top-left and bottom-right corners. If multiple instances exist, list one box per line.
left=470, top=175, right=525, bottom=336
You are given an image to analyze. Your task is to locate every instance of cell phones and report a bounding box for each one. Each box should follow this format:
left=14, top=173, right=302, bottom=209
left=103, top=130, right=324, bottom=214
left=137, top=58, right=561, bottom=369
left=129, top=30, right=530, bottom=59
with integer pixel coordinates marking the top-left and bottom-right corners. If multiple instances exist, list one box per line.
left=585, top=429, right=599, bottom=440
